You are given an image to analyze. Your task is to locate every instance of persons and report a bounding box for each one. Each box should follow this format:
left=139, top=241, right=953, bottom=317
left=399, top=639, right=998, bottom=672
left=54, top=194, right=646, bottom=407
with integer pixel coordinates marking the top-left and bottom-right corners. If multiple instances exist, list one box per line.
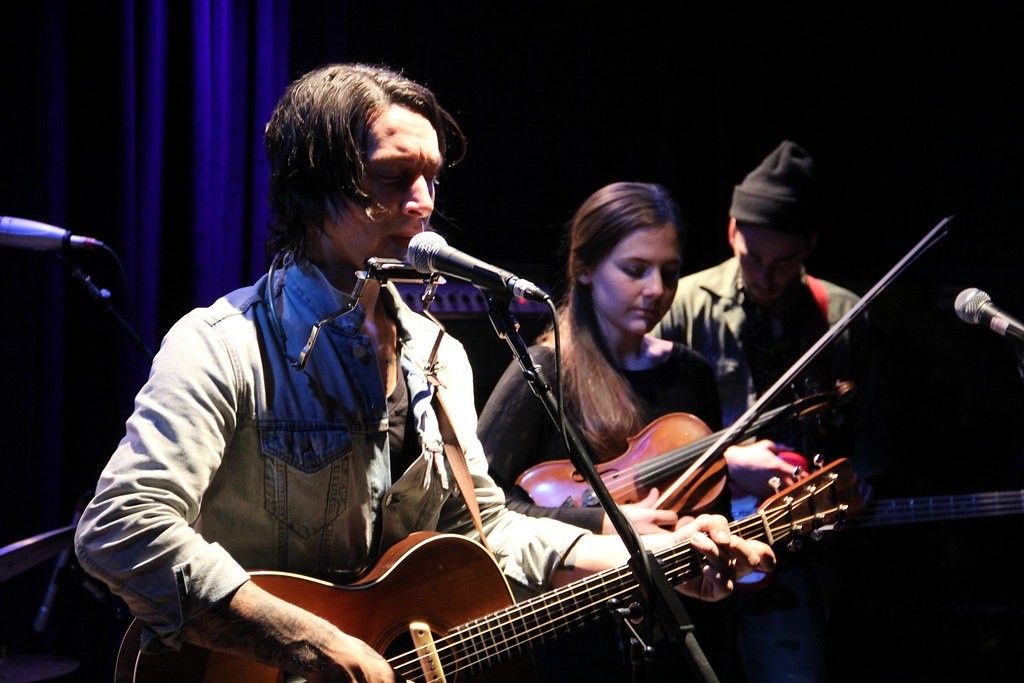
left=644, top=140, right=872, bottom=683
left=71, top=63, right=778, bottom=683
left=478, top=180, right=736, bottom=683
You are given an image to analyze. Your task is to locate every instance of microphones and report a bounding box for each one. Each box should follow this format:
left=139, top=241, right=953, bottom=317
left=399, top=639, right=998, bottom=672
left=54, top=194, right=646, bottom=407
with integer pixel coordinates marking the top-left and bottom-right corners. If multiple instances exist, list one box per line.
left=954, top=287, right=1024, bottom=342
left=0, top=215, right=104, bottom=253
left=408, top=230, right=550, bottom=304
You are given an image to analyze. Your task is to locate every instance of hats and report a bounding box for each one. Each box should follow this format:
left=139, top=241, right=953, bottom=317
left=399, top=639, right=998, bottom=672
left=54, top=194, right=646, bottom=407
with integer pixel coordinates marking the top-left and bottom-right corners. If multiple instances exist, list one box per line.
left=727, top=140, right=815, bottom=237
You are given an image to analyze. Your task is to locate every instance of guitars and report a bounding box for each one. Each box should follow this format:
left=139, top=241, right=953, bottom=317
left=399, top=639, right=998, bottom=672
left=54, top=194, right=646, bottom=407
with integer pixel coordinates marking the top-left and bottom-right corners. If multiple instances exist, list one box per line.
left=731, top=446, right=1024, bottom=591
left=112, top=456, right=867, bottom=683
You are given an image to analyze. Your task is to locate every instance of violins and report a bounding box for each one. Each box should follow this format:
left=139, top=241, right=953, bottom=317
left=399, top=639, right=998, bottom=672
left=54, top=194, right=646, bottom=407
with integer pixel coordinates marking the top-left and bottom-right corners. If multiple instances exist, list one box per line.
left=515, top=377, right=862, bottom=592
